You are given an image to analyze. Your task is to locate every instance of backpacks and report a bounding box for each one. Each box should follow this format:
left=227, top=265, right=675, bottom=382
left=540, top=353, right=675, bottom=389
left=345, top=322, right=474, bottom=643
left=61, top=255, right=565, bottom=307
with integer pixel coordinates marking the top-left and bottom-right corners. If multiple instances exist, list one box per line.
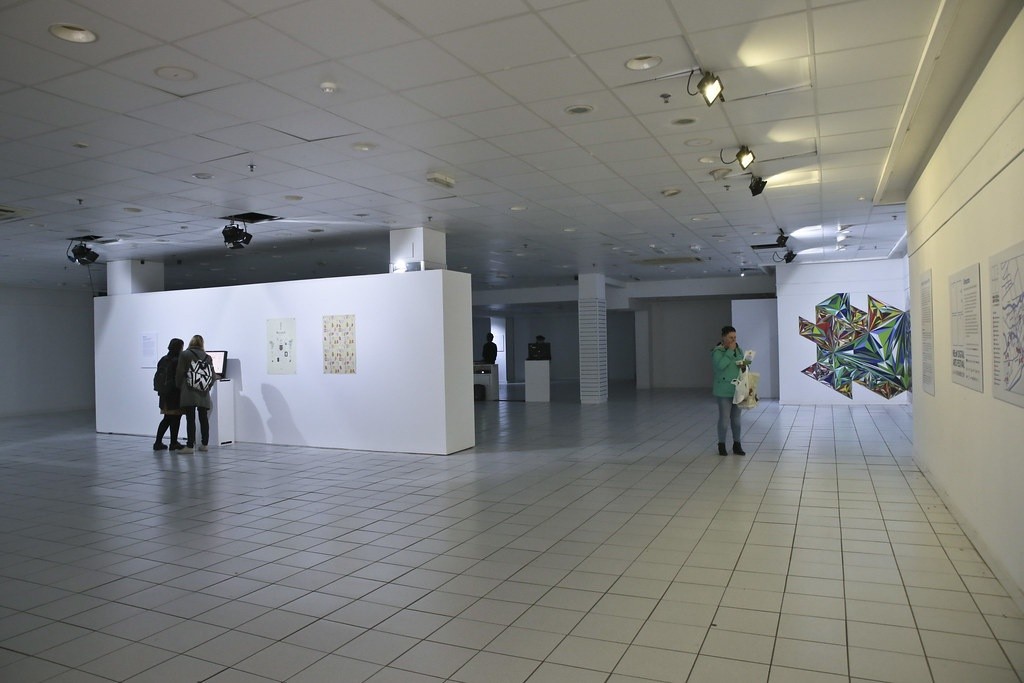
left=153, top=353, right=178, bottom=396
left=185, top=348, right=214, bottom=391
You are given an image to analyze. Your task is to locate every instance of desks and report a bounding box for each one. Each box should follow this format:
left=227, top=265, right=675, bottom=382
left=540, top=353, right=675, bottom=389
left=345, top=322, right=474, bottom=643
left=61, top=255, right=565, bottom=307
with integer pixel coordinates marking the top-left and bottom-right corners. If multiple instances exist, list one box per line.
left=474, top=364, right=498, bottom=402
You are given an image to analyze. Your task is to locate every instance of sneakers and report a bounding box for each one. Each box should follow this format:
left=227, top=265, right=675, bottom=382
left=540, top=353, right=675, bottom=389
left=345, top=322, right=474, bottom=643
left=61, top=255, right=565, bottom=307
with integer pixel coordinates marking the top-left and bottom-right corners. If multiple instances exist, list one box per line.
left=175, top=446, right=194, bottom=453
left=198, top=444, right=208, bottom=451
left=153, top=443, right=168, bottom=450
left=169, top=441, right=186, bottom=450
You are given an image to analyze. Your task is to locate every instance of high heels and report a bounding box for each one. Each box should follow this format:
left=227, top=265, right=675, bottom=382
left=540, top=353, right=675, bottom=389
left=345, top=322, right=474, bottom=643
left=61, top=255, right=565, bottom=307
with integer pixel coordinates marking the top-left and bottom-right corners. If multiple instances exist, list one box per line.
left=718, top=442, right=727, bottom=456
left=732, top=440, right=745, bottom=455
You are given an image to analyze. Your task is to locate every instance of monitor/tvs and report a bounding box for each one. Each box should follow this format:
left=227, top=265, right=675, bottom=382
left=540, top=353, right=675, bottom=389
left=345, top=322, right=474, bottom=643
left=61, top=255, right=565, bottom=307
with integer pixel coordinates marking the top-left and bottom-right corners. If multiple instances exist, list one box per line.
left=205, top=351, right=227, bottom=379
left=528, top=343, right=550, bottom=360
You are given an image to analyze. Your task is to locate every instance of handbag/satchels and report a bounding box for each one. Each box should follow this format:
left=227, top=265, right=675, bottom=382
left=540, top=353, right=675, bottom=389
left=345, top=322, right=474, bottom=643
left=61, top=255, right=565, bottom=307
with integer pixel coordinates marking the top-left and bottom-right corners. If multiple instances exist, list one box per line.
left=730, top=365, right=749, bottom=404
left=736, top=364, right=760, bottom=409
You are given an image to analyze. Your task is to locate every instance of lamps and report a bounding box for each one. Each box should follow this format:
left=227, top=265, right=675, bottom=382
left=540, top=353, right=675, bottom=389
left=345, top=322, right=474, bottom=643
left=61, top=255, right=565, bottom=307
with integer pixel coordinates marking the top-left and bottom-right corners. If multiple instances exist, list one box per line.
left=222, top=216, right=253, bottom=249
left=66, top=237, right=100, bottom=265
left=720, top=145, right=757, bottom=170
left=687, top=69, right=724, bottom=107
left=776, top=232, right=789, bottom=248
left=749, top=176, right=767, bottom=197
left=773, top=250, right=797, bottom=264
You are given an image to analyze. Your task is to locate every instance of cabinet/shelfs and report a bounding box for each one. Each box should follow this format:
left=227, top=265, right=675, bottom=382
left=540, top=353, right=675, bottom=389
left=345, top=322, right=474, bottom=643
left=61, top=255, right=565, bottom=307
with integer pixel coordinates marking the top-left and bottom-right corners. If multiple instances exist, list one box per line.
left=195, top=379, right=235, bottom=448
left=524, top=360, right=550, bottom=402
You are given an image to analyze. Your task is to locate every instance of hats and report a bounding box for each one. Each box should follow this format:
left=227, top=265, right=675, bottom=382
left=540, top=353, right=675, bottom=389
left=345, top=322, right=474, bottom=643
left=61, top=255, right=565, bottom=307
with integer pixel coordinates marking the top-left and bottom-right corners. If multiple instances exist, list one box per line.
left=536, top=335, right=546, bottom=341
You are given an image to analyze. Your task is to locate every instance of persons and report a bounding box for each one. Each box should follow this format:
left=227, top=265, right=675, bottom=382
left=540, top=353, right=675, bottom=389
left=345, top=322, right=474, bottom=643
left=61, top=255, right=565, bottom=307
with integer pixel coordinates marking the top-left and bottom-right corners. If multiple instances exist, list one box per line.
left=711, top=326, right=750, bottom=456
left=482, top=332, right=497, bottom=364
left=175, top=335, right=216, bottom=453
left=153, top=338, right=185, bottom=450
